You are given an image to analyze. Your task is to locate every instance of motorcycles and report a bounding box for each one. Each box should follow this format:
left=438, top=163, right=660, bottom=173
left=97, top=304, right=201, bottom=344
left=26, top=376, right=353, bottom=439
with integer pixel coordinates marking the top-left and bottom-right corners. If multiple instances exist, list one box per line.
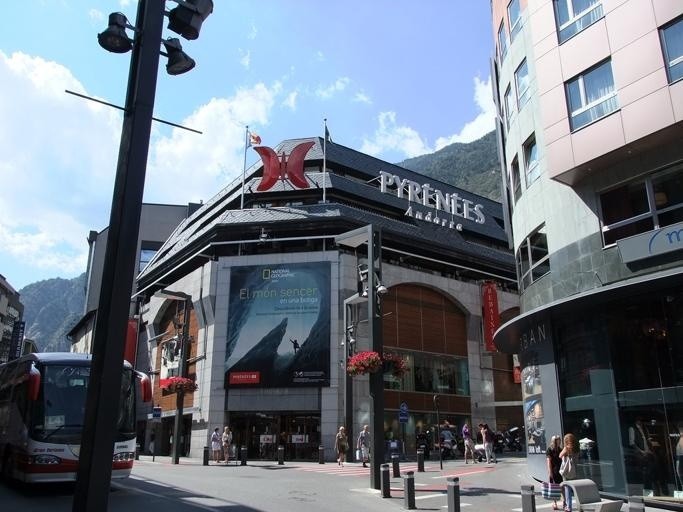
left=388, top=424, right=523, bottom=460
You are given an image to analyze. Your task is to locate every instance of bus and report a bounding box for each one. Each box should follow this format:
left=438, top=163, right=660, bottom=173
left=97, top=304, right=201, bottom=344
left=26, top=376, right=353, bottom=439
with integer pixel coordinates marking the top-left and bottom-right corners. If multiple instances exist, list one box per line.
left=0, top=351, right=152, bottom=485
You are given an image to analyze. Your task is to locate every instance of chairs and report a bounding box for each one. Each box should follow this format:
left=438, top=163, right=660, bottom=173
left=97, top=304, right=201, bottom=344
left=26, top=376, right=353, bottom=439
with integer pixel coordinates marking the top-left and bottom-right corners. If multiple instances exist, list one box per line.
left=560, top=479, right=624, bottom=512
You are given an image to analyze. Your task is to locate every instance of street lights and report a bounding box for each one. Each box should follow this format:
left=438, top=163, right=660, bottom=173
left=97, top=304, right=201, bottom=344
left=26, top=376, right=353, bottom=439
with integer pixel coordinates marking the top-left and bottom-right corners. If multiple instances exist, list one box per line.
left=70, top=0, right=213, bottom=512
left=340, top=291, right=367, bottom=462
left=153, top=288, right=192, bottom=466
left=433, top=394, right=443, bottom=470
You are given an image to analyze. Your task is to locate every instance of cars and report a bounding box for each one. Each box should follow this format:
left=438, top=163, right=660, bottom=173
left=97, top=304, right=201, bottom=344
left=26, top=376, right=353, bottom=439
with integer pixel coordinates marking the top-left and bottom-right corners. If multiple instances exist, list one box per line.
left=251, top=412, right=319, bottom=463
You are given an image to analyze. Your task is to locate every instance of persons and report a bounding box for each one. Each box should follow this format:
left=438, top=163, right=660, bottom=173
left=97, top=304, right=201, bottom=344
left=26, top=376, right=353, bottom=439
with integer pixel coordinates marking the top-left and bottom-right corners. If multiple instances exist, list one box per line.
left=558, top=433, right=580, bottom=512
left=221, top=426, right=233, bottom=463
left=334, top=426, right=350, bottom=467
left=545, top=435, right=567, bottom=511
left=440, top=425, right=454, bottom=440
left=290, top=339, right=300, bottom=353
left=628, top=416, right=649, bottom=457
left=675, top=428, right=683, bottom=486
left=211, top=427, right=222, bottom=463
left=357, top=425, right=370, bottom=468
left=462, top=423, right=497, bottom=464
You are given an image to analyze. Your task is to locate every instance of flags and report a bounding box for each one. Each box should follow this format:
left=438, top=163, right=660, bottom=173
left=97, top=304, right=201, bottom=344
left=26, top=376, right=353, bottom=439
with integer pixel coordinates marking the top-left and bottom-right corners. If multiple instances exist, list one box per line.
left=248, top=132, right=261, bottom=146
left=326, top=125, right=334, bottom=145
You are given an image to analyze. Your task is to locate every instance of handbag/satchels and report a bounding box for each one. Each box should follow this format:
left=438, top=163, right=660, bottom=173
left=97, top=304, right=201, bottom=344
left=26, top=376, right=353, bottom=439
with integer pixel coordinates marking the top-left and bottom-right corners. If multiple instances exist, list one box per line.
left=542, top=482, right=561, bottom=500
